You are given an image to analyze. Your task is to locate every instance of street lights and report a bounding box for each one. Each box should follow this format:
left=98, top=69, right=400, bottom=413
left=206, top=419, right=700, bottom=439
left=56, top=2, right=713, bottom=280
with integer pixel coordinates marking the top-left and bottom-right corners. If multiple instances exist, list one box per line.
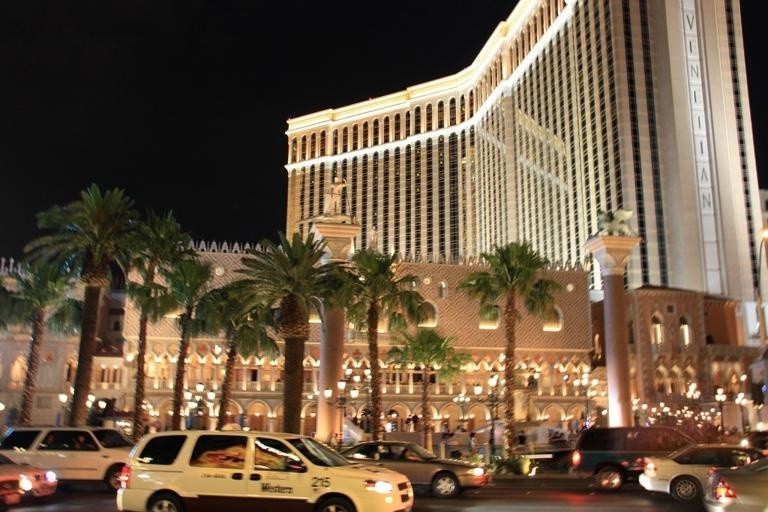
left=471, top=373, right=508, bottom=454
left=714, top=387, right=725, bottom=438
left=182, top=382, right=217, bottom=430
left=322, top=380, right=359, bottom=451
left=734, top=392, right=750, bottom=435
left=84, top=399, right=107, bottom=427
left=59, top=385, right=95, bottom=426
left=453, top=393, right=471, bottom=431
left=572, top=372, right=600, bottom=429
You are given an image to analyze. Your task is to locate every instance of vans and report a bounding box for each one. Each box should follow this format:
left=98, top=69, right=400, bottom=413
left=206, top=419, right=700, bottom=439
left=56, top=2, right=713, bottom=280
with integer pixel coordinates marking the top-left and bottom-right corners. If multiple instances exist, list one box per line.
left=0, top=423, right=140, bottom=492
left=111, top=428, right=417, bottom=512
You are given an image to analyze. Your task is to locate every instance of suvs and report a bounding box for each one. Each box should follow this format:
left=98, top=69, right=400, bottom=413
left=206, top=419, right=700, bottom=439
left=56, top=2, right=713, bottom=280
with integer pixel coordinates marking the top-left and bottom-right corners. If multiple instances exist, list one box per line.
left=568, top=424, right=699, bottom=494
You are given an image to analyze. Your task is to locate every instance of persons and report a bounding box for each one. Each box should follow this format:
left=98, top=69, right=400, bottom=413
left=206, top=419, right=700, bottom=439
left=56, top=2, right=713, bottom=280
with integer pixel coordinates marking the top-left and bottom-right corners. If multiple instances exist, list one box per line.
left=519, top=430, right=526, bottom=445
left=330, top=433, right=343, bottom=449
left=327, top=176, right=346, bottom=213
left=47, top=434, right=54, bottom=445
left=406, top=413, right=418, bottom=432
left=73, top=435, right=85, bottom=450
left=440, top=432, right=458, bottom=458
left=470, top=432, right=477, bottom=454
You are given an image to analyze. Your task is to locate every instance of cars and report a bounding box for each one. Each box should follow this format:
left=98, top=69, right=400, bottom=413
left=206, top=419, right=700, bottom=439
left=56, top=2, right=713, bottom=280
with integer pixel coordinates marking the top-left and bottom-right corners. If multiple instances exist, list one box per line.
left=1, top=452, right=60, bottom=505
left=330, top=440, right=496, bottom=500
left=698, top=461, right=768, bottom=511
left=634, top=443, right=768, bottom=503
